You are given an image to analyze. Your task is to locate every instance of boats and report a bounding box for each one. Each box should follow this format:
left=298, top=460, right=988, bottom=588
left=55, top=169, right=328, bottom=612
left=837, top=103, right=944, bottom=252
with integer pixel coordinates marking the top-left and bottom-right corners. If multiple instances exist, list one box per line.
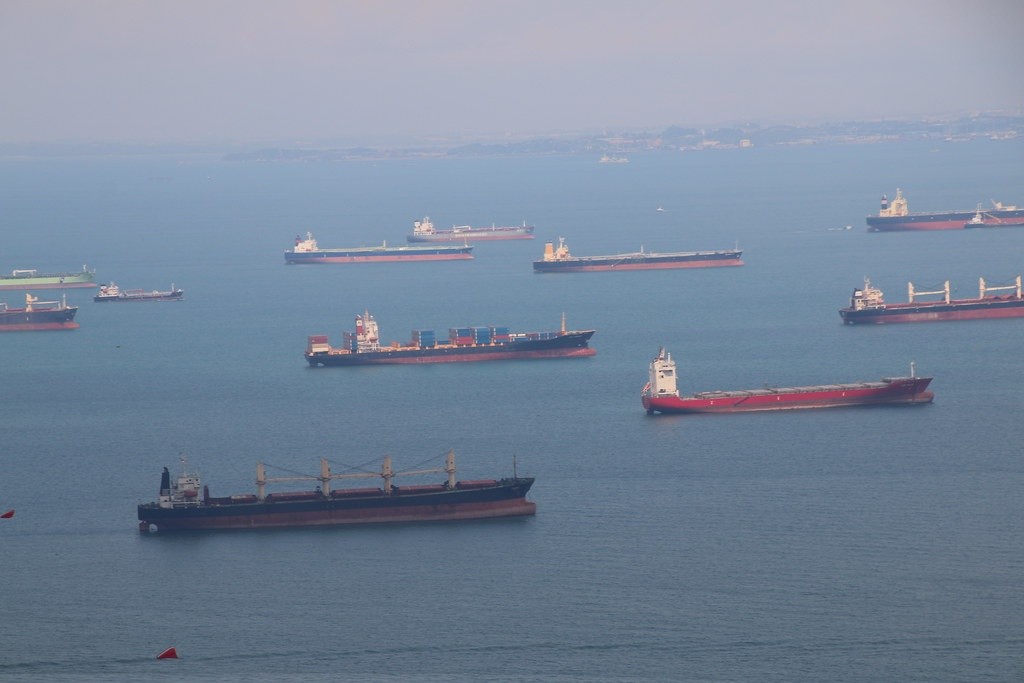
left=280, top=233, right=473, bottom=261
left=530, top=236, right=748, bottom=271
left=405, top=218, right=537, bottom=241
left=0, top=293, right=81, bottom=329
left=864, top=188, right=1024, bottom=233
left=640, top=349, right=935, bottom=415
left=839, top=278, right=1022, bottom=325
left=0, top=265, right=99, bottom=289
left=92, top=282, right=183, bottom=304
left=134, top=465, right=539, bottom=535
left=303, top=309, right=599, bottom=367
